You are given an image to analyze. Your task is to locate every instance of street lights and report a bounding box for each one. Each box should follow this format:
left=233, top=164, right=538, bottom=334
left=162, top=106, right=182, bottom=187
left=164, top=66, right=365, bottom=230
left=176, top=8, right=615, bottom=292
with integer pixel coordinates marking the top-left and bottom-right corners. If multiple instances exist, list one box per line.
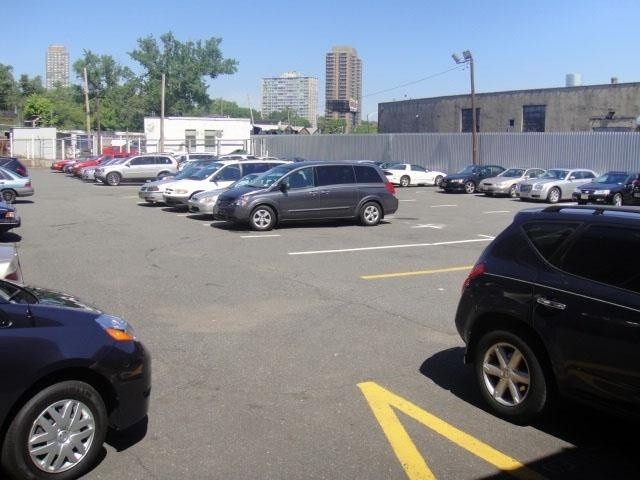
left=453, top=49, right=478, bottom=166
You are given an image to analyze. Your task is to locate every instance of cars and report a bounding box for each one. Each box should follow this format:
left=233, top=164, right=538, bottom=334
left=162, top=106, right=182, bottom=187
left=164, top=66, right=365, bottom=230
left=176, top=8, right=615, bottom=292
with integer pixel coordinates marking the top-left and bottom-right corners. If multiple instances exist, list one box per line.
left=0, top=156, right=33, bottom=283
left=384, top=161, right=447, bottom=188
left=438, top=163, right=640, bottom=206
left=0, top=279, right=152, bottom=480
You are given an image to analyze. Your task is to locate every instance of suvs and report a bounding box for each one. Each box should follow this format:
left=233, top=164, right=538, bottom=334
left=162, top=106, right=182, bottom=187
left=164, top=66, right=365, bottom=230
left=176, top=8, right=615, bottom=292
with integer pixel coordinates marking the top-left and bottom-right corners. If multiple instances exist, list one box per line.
left=452, top=205, right=639, bottom=418
